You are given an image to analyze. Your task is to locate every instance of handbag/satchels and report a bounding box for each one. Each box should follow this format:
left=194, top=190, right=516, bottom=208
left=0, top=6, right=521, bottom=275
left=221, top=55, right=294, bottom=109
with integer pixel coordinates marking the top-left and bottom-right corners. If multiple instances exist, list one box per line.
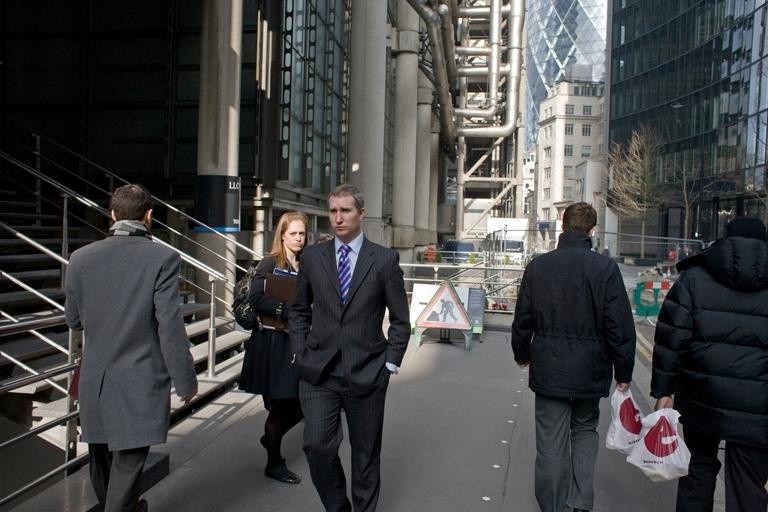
left=232, top=259, right=263, bottom=330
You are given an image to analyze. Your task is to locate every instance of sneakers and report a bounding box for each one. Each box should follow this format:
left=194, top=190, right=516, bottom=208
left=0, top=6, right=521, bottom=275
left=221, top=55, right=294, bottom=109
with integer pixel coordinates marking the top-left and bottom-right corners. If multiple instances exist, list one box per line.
left=265, top=458, right=302, bottom=483
left=134, top=498, right=147, bottom=512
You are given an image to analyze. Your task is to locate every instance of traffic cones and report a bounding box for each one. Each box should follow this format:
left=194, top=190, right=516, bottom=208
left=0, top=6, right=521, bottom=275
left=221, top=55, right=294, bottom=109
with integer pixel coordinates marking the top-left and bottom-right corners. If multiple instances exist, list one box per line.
left=427, top=241, right=435, bottom=262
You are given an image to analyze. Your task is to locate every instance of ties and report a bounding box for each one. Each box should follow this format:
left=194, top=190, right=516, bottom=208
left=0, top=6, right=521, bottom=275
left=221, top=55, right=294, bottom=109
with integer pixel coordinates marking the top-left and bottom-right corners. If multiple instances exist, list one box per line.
left=337, top=243, right=353, bottom=306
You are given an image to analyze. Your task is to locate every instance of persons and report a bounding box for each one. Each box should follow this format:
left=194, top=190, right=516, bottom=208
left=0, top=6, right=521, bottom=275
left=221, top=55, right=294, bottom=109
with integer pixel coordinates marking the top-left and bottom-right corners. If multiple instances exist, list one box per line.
left=290, top=183, right=411, bottom=510
left=61, top=183, right=198, bottom=510
left=647, top=213, right=768, bottom=511
left=238, top=210, right=318, bottom=483
left=510, top=200, right=638, bottom=512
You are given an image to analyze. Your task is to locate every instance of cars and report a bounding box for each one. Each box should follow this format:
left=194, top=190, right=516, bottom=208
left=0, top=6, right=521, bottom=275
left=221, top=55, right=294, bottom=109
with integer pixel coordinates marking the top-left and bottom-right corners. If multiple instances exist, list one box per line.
left=525, top=252, right=547, bottom=267
left=440, top=239, right=475, bottom=263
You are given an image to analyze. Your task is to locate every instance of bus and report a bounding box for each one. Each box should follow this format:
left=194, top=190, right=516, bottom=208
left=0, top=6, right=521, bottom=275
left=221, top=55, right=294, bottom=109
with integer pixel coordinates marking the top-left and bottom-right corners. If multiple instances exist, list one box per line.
left=495, top=239, right=523, bottom=264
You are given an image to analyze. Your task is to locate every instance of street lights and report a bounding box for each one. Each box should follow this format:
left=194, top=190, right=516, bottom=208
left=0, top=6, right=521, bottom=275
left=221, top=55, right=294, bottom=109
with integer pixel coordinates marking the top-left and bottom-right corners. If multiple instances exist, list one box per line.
left=631, top=176, right=647, bottom=259
left=664, top=180, right=700, bottom=239
